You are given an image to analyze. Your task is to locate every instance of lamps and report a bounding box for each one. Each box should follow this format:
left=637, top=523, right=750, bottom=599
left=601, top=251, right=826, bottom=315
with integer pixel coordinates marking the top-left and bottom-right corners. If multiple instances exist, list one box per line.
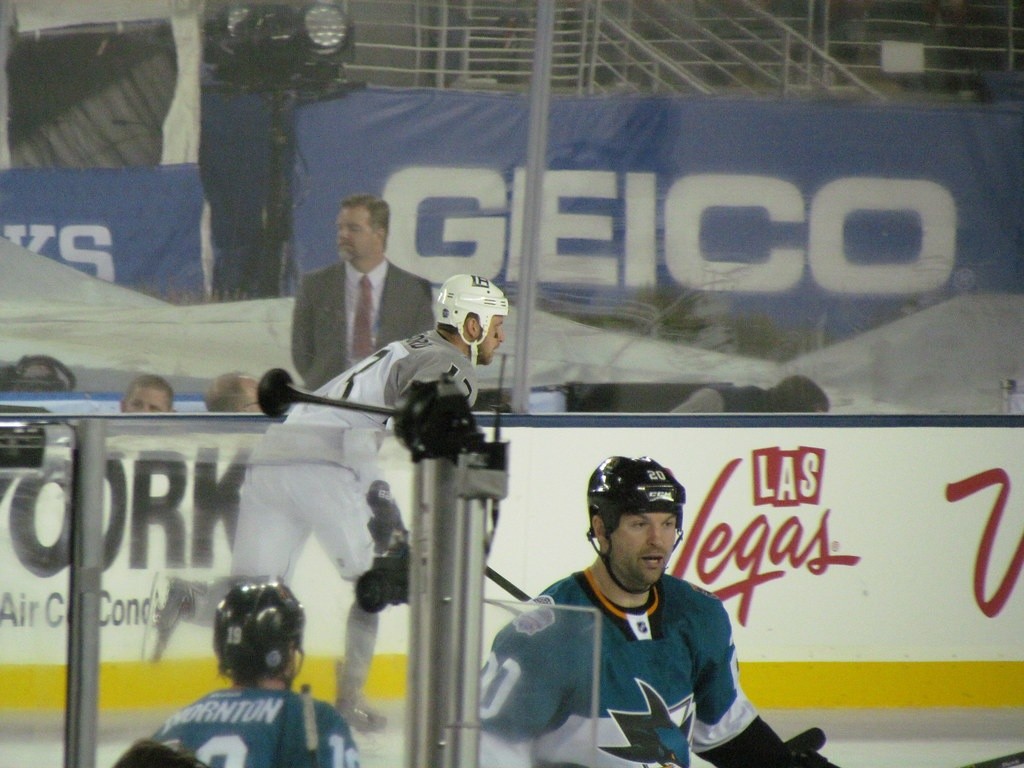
left=204, top=0, right=355, bottom=80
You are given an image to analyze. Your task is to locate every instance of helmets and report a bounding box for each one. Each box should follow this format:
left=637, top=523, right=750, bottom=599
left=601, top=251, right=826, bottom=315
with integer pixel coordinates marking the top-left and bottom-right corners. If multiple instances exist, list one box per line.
left=588, top=456, right=686, bottom=536
left=212, top=581, right=304, bottom=684
left=433, top=274, right=508, bottom=336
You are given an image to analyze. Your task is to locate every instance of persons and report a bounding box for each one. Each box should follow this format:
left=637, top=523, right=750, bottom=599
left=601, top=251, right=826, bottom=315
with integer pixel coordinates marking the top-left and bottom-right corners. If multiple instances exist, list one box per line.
left=120, top=376, right=176, bottom=414
left=478, top=456, right=836, bottom=767
left=204, top=372, right=262, bottom=414
left=230, top=275, right=509, bottom=734
left=290, top=192, right=435, bottom=392
left=669, top=375, right=829, bottom=413
left=151, top=583, right=362, bottom=768
left=112, top=737, right=203, bottom=768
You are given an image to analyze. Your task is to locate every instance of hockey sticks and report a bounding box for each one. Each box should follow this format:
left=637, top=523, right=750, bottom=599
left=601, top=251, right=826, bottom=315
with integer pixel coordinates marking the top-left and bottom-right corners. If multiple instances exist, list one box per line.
left=962, top=747, right=1024, bottom=768
left=486, top=563, right=535, bottom=607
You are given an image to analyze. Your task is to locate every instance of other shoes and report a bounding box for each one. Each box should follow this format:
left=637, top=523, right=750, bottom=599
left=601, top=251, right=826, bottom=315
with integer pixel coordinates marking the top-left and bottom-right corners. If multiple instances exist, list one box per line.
left=142, top=570, right=207, bottom=665
left=333, top=694, right=389, bottom=752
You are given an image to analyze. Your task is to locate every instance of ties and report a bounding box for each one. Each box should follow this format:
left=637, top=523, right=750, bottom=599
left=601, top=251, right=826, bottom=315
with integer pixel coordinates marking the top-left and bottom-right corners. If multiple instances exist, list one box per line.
left=352, top=274, right=373, bottom=367
